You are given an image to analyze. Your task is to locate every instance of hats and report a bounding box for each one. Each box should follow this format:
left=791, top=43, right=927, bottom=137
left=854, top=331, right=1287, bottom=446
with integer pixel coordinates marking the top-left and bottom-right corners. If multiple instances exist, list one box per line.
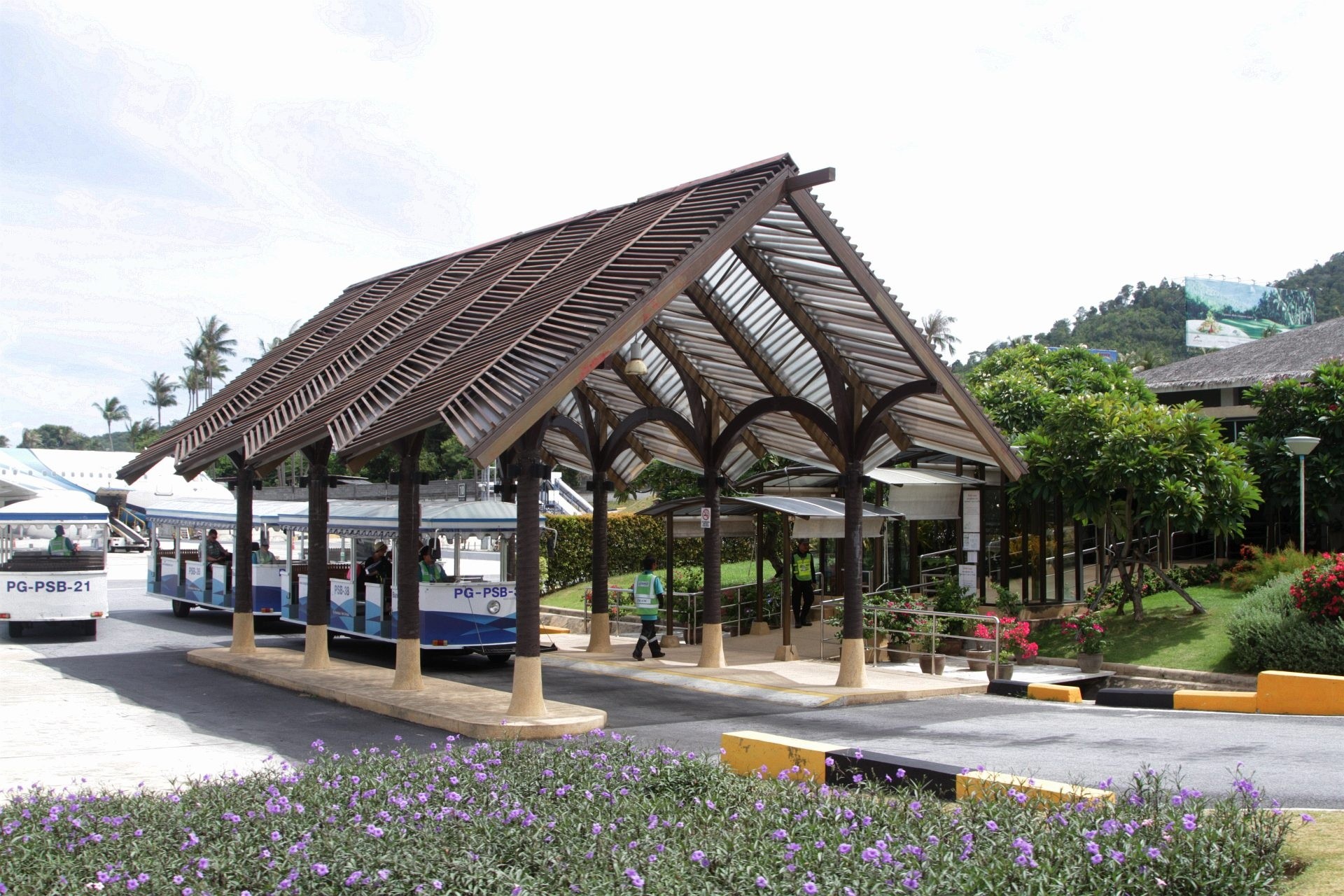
left=56, top=525, right=65, bottom=535
left=262, top=539, right=269, bottom=545
left=800, top=538, right=810, bottom=543
left=374, top=542, right=385, bottom=552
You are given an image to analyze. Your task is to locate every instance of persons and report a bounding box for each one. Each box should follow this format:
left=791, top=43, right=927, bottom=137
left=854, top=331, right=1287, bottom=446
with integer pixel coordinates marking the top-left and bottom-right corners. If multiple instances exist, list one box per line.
left=198, top=529, right=233, bottom=593
left=365, top=542, right=392, bottom=584
left=252, top=540, right=276, bottom=564
left=418, top=546, right=448, bottom=583
left=631, top=555, right=665, bottom=661
left=132, top=521, right=141, bottom=533
left=790, top=539, right=817, bottom=629
left=47, top=525, right=77, bottom=556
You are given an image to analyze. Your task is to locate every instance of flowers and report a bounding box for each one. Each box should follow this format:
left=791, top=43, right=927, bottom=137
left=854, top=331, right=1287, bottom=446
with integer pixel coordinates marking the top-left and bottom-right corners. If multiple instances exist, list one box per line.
left=991, top=621, right=1038, bottom=665
left=662, top=563, right=704, bottom=628
left=580, top=584, right=635, bottom=620
left=1061, top=605, right=1106, bottom=655
left=822, top=590, right=932, bottom=643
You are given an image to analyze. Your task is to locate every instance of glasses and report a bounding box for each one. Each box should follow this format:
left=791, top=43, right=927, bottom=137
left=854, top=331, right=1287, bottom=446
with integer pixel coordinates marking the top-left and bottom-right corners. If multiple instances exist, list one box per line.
left=212, top=537, right=216, bottom=539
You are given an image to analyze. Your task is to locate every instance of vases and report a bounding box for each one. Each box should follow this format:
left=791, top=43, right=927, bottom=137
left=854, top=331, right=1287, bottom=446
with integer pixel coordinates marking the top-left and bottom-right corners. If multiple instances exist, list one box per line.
left=965, top=648, right=991, bottom=672
left=887, top=642, right=911, bottom=662
left=1076, top=653, right=1103, bottom=672
left=683, top=628, right=703, bottom=644
left=986, top=660, right=1014, bottom=681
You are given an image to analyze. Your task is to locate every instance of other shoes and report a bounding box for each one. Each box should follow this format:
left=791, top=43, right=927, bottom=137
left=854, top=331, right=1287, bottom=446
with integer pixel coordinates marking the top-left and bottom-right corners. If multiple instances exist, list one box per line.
left=227, top=590, right=232, bottom=593
left=633, top=654, right=644, bottom=661
left=795, top=622, right=801, bottom=628
left=801, top=622, right=812, bottom=626
left=653, top=652, right=665, bottom=657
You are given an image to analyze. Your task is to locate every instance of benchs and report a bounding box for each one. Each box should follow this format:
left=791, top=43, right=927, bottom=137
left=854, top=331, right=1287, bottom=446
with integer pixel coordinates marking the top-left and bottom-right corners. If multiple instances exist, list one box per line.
left=0, top=551, right=105, bottom=572
left=147, top=549, right=391, bottom=637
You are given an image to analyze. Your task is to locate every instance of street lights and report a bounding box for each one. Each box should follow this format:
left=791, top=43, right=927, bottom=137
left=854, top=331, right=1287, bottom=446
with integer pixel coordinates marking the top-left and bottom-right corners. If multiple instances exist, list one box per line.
left=1284, top=437, right=1322, bottom=558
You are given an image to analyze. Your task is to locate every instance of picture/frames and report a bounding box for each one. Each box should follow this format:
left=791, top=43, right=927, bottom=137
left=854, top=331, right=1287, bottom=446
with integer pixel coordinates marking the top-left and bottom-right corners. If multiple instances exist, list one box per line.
left=973, top=611, right=1016, bottom=652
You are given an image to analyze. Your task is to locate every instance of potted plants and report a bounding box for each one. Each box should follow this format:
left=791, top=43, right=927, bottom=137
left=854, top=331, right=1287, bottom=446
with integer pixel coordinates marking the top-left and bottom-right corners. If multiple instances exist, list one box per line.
left=991, top=580, right=1037, bottom=665
left=924, top=572, right=978, bottom=653
left=917, top=613, right=951, bottom=675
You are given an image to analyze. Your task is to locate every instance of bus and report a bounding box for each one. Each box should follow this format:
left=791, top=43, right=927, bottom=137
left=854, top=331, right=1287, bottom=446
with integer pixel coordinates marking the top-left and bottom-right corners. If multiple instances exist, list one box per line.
left=144, top=500, right=558, bottom=669
left=0, top=491, right=112, bottom=637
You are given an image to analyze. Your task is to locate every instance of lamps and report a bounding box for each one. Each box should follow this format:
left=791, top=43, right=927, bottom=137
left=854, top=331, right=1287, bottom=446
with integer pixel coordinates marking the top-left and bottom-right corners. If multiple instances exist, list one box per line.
left=229, top=463, right=615, bottom=492
left=624, top=332, right=649, bottom=376
left=838, top=474, right=871, bottom=490
left=697, top=475, right=729, bottom=491
